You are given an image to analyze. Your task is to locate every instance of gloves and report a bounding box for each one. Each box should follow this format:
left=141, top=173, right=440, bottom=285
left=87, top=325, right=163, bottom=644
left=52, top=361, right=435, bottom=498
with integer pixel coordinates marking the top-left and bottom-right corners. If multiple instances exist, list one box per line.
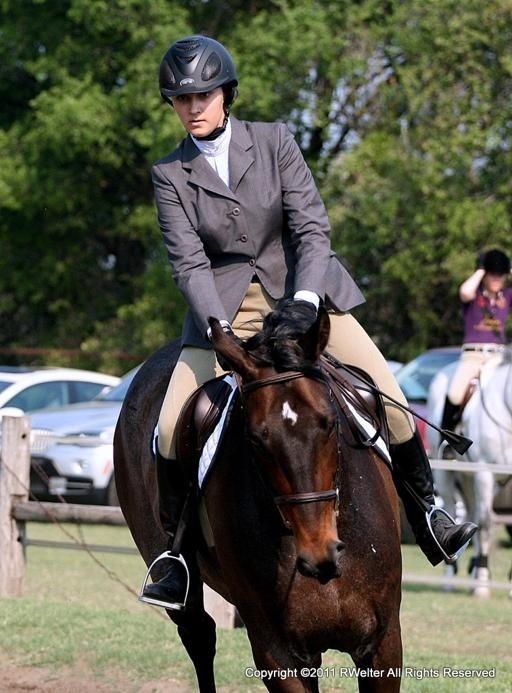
left=209, top=329, right=247, bottom=371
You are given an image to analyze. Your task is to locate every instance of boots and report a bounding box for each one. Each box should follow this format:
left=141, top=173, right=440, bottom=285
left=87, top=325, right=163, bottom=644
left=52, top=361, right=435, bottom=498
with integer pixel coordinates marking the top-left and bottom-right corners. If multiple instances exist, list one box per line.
left=388, top=424, right=478, bottom=565
left=438, top=394, right=461, bottom=459
left=144, top=435, right=187, bottom=605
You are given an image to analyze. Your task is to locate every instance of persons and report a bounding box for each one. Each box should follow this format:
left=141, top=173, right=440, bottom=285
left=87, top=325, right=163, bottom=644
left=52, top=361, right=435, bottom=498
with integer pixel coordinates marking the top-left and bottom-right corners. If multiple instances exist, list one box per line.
left=438, top=249, right=512, bottom=459
left=143, top=36, right=480, bottom=604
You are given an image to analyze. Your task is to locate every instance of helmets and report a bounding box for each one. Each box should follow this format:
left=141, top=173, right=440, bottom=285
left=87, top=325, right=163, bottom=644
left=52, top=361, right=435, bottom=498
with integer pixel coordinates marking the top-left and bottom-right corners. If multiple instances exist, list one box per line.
left=159, top=34, right=240, bottom=97
left=473, top=249, right=511, bottom=276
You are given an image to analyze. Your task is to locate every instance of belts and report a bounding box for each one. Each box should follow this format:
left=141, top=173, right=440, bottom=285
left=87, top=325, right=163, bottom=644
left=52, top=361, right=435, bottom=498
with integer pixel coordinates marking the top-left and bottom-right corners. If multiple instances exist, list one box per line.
left=464, top=347, right=493, bottom=351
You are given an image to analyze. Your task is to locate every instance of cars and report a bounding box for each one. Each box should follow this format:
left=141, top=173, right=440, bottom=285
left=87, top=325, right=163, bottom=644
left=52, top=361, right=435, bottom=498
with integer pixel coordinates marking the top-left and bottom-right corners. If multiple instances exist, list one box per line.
left=391, top=344, right=511, bottom=456
left=0, top=364, right=153, bottom=505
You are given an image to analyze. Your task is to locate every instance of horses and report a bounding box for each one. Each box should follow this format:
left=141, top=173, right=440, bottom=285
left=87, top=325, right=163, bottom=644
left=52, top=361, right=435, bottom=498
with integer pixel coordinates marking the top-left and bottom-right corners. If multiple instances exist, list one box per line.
left=424, top=356, right=511, bottom=602
left=111, top=295, right=403, bottom=693
